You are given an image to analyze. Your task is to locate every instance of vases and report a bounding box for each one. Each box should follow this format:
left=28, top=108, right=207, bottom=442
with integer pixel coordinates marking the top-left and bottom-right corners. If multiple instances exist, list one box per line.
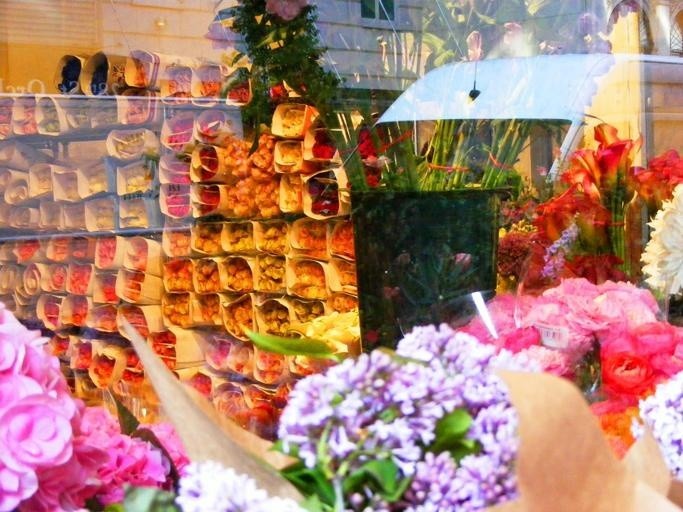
left=345, top=186, right=516, bottom=354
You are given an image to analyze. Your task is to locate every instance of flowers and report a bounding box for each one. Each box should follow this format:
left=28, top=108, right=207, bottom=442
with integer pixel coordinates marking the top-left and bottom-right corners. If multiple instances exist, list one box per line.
left=0, top=0, right=683, bottom=512
